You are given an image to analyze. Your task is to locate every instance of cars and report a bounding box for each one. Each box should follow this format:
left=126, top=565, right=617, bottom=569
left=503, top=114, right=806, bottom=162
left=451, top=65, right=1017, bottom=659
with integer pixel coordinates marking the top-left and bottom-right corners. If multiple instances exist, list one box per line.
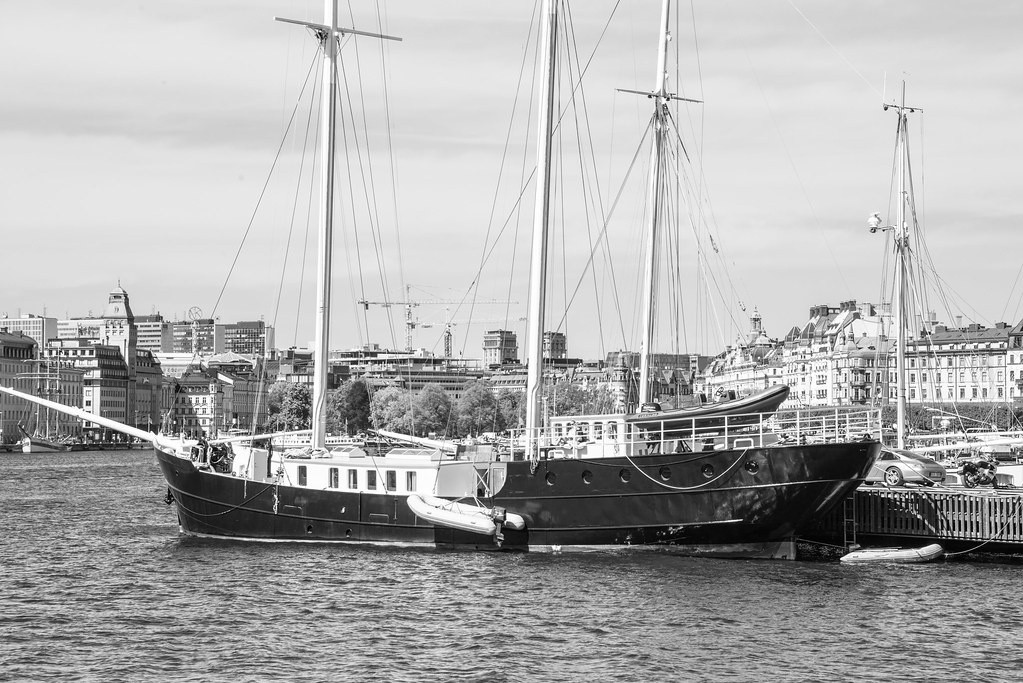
left=862, top=448, right=946, bottom=488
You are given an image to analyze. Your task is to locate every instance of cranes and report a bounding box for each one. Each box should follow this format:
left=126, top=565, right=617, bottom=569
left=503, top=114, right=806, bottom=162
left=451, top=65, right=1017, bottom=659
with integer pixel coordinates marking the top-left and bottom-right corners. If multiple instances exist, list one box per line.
left=352, top=296, right=529, bottom=358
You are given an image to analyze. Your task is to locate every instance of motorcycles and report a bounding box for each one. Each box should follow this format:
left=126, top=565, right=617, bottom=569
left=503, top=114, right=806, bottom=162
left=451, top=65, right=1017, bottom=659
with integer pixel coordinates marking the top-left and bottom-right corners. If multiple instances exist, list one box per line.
left=956, top=449, right=999, bottom=488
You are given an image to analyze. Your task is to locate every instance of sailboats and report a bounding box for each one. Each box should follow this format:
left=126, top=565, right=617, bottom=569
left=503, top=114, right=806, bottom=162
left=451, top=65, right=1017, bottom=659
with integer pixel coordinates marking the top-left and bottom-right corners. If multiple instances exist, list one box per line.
left=145, top=2, right=513, bottom=548
left=17, top=341, right=70, bottom=455
left=406, top=0, right=1020, bottom=555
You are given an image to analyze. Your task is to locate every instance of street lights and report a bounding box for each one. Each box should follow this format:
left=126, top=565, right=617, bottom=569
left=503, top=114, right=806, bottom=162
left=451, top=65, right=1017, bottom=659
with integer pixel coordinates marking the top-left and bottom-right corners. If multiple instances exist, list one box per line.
left=135, top=410, right=139, bottom=429
left=102, top=409, right=108, bottom=436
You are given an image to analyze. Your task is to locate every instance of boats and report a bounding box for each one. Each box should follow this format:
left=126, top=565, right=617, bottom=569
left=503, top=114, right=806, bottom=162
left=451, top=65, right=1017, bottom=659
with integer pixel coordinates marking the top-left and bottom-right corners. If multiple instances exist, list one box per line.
left=622, top=380, right=793, bottom=436
left=840, top=541, right=942, bottom=567
